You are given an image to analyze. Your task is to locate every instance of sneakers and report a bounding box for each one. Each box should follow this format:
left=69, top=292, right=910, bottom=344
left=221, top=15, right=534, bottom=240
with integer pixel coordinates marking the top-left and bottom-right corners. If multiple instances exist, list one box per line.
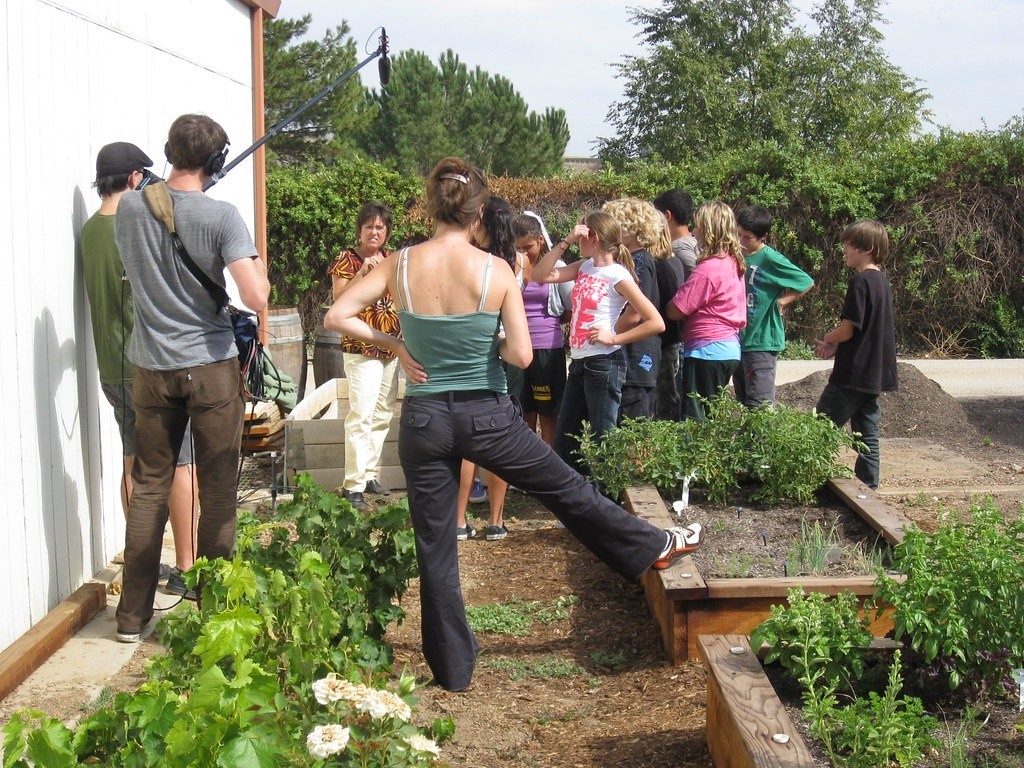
left=469, top=481, right=487, bottom=503
left=457, top=523, right=476, bottom=541
left=159, top=564, right=196, bottom=600
left=653, top=523, right=705, bottom=569
left=486, top=522, right=508, bottom=540
left=115, top=625, right=149, bottom=642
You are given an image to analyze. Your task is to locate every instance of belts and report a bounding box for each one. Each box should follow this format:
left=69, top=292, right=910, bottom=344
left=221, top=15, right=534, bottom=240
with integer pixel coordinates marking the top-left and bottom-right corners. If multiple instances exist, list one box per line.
left=410, top=389, right=498, bottom=403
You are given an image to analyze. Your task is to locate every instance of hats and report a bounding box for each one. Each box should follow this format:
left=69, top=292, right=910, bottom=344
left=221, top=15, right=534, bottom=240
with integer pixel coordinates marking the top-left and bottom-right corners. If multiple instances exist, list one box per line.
left=96, top=142, right=153, bottom=174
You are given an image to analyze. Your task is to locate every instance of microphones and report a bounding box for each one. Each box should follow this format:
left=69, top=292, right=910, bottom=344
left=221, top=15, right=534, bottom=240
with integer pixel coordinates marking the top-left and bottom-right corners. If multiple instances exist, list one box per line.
left=379, top=27, right=391, bottom=85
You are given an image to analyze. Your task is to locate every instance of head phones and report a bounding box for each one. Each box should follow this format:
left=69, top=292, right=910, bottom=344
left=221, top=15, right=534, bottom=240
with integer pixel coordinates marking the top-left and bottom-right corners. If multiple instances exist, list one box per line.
left=165, top=129, right=230, bottom=176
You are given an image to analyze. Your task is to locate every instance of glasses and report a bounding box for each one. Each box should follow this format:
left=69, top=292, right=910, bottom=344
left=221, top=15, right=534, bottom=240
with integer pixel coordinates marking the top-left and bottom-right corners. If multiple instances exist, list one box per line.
left=129, top=169, right=150, bottom=179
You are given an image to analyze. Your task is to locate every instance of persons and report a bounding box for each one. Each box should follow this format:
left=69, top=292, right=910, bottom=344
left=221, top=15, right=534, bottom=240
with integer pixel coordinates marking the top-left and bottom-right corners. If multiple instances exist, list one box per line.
left=814, top=218, right=899, bottom=490
left=457, top=189, right=814, bottom=542
left=328, top=199, right=400, bottom=510
left=80, top=113, right=271, bottom=642
left=324, top=154, right=703, bottom=692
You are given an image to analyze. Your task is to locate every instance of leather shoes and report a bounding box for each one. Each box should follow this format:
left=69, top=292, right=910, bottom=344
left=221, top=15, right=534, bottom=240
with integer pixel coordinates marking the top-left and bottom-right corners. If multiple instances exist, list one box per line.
left=341, top=487, right=367, bottom=508
left=366, top=479, right=391, bottom=495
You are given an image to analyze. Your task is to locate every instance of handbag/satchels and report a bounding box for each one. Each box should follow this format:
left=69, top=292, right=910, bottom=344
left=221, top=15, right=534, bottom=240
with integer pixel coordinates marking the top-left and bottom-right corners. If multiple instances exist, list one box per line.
left=228, top=304, right=261, bottom=361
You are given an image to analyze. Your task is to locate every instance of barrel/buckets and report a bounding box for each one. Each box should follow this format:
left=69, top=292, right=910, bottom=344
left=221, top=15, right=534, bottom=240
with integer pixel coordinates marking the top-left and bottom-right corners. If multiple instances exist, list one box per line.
left=268, top=305, right=308, bottom=406
left=313, top=306, right=347, bottom=390
